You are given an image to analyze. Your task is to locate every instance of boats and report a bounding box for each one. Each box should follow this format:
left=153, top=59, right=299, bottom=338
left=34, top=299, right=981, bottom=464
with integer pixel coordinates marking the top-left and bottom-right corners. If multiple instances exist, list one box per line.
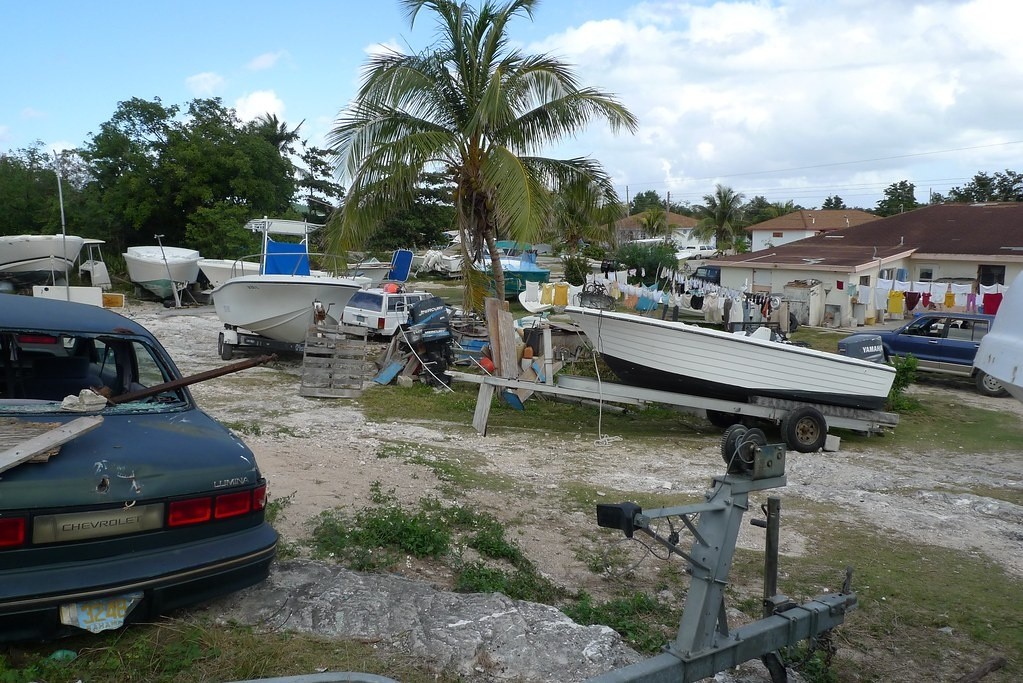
left=198, top=258, right=394, bottom=296
left=411, top=230, right=496, bottom=273
left=567, top=306, right=896, bottom=413
left=210, top=215, right=362, bottom=345
left=470, top=256, right=550, bottom=300
left=121, top=243, right=204, bottom=297
left=0, top=235, right=84, bottom=280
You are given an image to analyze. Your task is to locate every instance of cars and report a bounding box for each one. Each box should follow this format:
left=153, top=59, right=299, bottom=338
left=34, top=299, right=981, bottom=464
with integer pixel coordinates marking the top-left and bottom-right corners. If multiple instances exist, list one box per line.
left=836, top=311, right=1013, bottom=397
left=678, top=265, right=721, bottom=292
left=0, top=291, right=281, bottom=643
left=684, top=245, right=718, bottom=260
left=340, top=289, right=434, bottom=339
